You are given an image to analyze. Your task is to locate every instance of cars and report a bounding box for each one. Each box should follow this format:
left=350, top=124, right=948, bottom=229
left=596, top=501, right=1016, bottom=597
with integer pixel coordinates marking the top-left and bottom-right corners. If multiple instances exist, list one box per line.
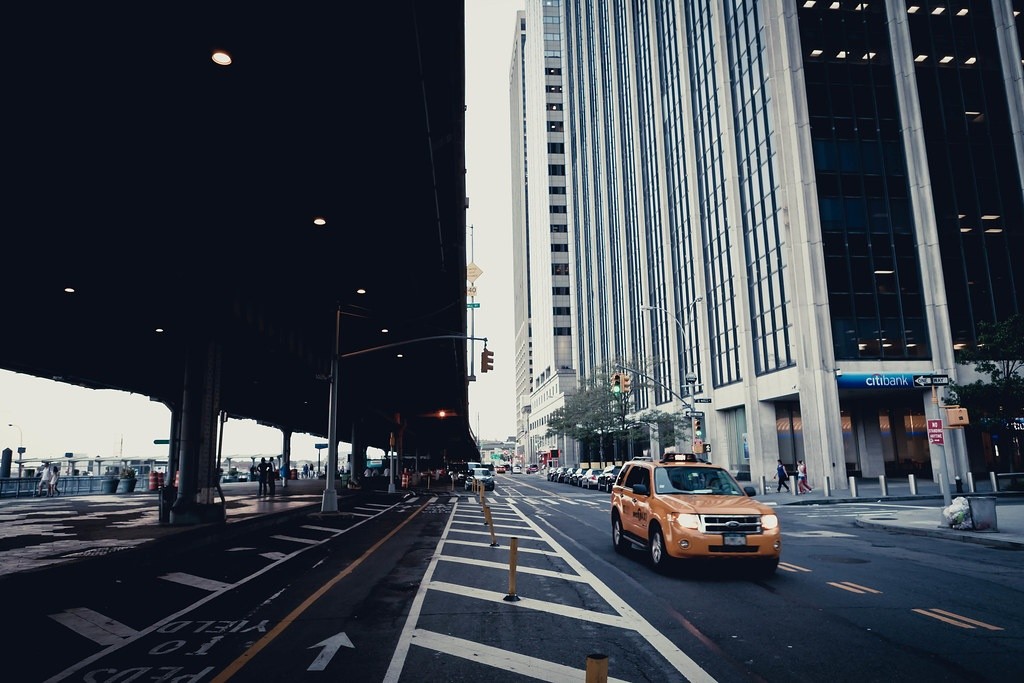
left=527, top=464, right=539, bottom=474
left=420, top=458, right=523, bottom=491
left=547, top=466, right=590, bottom=487
left=582, top=469, right=604, bottom=489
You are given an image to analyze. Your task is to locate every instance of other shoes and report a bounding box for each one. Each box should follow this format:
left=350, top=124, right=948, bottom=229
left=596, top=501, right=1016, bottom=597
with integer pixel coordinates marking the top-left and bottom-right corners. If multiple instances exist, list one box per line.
left=776, top=488, right=812, bottom=494
left=35, top=492, right=61, bottom=498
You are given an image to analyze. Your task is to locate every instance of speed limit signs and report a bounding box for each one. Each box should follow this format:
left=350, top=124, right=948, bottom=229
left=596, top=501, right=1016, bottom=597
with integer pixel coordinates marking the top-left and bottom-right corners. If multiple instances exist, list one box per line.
left=466, top=286, right=476, bottom=297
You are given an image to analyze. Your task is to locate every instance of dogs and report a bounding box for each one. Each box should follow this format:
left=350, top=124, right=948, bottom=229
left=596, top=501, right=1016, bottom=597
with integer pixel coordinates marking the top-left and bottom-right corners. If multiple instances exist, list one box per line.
left=766, top=485, right=772, bottom=493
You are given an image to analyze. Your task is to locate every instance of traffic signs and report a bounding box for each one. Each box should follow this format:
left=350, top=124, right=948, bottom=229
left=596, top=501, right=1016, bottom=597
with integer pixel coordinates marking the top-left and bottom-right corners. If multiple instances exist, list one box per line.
left=686, top=411, right=704, bottom=417
left=913, top=374, right=950, bottom=387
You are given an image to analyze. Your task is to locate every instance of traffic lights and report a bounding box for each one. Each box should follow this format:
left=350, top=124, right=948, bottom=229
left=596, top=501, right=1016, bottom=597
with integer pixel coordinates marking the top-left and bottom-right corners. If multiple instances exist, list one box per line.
left=613, top=373, right=620, bottom=393
left=481, top=350, right=494, bottom=373
left=703, top=443, right=712, bottom=452
left=619, top=374, right=630, bottom=393
left=694, top=419, right=702, bottom=438
left=610, top=375, right=614, bottom=393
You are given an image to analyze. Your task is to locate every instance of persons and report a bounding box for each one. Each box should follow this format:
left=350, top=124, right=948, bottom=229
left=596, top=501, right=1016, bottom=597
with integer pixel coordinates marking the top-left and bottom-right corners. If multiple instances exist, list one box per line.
left=249, top=456, right=390, bottom=496
left=36, top=462, right=61, bottom=498
left=773, top=459, right=791, bottom=493
left=454, top=466, right=458, bottom=478
left=795, top=459, right=812, bottom=494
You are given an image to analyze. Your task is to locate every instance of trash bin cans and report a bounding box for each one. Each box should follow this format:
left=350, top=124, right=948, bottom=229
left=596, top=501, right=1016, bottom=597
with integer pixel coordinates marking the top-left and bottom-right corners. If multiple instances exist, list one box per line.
left=159, top=486, right=178, bottom=522
left=967, top=496, right=1000, bottom=533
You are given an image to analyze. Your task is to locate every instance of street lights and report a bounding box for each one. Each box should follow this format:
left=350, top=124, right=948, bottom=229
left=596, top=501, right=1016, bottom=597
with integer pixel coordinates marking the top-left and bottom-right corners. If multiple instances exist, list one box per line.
left=640, top=296, right=705, bottom=412
left=8, top=424, right=22, bottom=477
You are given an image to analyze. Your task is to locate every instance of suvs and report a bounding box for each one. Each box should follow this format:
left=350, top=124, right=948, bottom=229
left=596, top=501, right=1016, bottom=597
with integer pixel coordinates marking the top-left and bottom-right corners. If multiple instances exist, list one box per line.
left=610, top=452, right=782, bottom=578
left=598, top=465, right=623, bottom=494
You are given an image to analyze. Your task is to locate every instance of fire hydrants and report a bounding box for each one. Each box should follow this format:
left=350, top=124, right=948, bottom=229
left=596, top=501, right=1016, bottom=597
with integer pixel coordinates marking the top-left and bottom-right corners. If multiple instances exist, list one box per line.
left=954, top=476, right=964, bottom=493
